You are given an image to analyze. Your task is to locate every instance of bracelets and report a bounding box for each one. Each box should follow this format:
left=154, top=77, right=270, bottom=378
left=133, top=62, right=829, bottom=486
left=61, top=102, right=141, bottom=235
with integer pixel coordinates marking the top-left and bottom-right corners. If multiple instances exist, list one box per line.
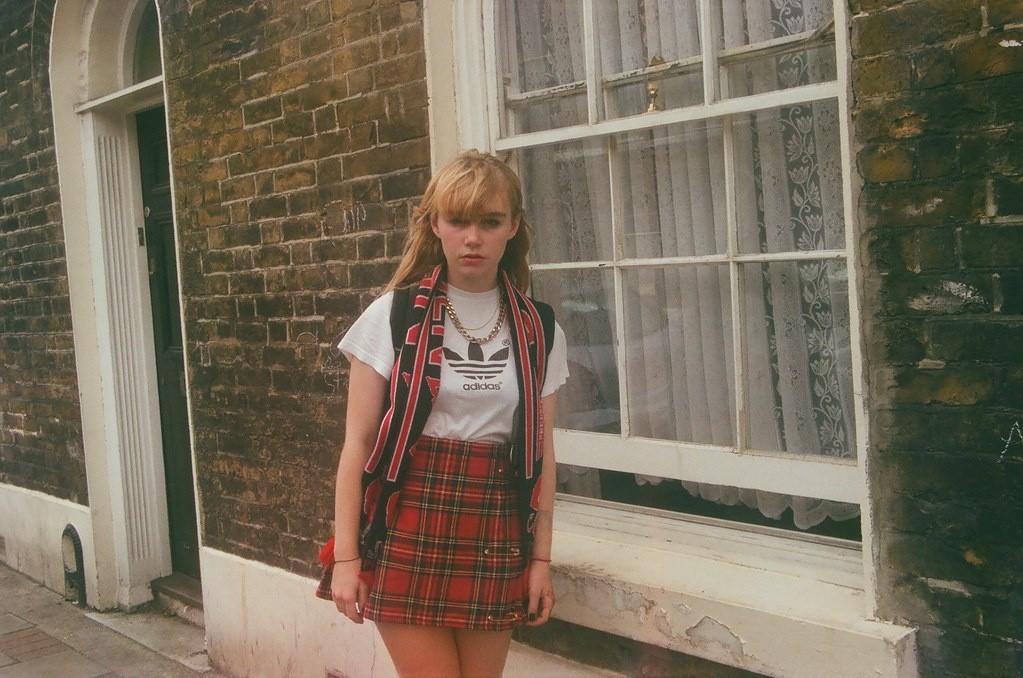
left=530, top=556, right=551, bottom=562
left=334, top=556, right=360, bottom=563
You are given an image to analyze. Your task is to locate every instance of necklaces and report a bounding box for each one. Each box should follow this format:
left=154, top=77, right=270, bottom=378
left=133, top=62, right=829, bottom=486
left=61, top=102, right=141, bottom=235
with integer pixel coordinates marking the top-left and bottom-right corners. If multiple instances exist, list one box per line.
left=445, top=280, right=507, bottom=345
left=461, top=303, right=499, bottom=330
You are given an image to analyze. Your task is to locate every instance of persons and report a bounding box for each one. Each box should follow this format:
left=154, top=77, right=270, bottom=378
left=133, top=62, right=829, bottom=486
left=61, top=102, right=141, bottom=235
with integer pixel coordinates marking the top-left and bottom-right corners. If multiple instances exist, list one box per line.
left=317, top=148, right=570, bottom=678
